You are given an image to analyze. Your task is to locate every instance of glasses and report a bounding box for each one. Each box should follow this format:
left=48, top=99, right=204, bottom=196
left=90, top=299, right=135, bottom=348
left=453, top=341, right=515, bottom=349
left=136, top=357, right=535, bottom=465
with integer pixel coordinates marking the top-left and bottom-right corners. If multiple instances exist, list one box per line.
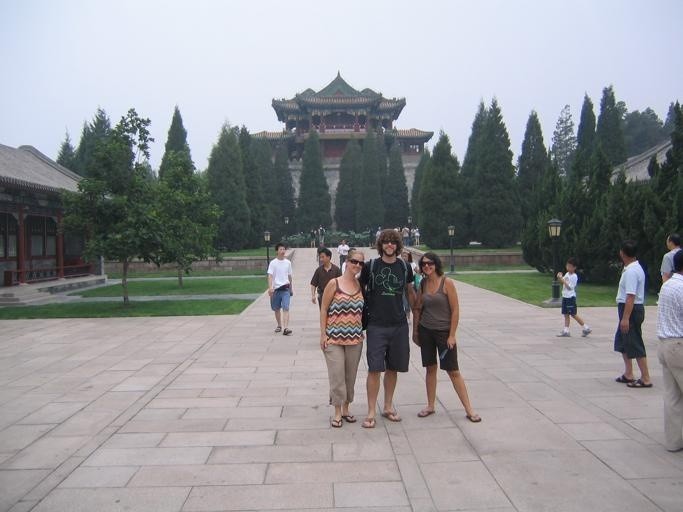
left=381, top=239, right=398, bottom=245
left=421, top=260, right=435, bottom=267
left=348, top=259, right=364, bottom=266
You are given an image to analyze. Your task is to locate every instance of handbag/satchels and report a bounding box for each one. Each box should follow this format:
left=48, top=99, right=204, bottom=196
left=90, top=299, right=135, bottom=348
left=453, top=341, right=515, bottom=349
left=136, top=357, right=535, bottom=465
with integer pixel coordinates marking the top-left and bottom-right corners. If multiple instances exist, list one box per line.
left=362, top=300, right=368, bottom=330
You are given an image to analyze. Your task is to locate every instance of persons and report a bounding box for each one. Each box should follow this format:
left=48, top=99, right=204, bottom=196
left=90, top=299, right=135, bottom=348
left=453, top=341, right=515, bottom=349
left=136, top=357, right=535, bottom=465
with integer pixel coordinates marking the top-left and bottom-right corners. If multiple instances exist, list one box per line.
left=656, top=250, right=683, bottom=452
left=310, top=223, right=421, bottom=324
left=359, top=229, right=417, bottom=428
left=660, top=233, right=683, bottom=282
left=413, top=253, right=482, bottom=423
left=556, top=257, right=592, bottom=336
left=319, top=250, right=365, bottom=428
left=612, top=240, right=653, bottom=388
left=267, top=243, right=294, bottom=334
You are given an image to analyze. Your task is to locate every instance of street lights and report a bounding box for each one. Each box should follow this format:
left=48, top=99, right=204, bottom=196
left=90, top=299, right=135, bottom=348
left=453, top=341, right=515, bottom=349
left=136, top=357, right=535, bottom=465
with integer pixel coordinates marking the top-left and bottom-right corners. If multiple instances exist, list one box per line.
left=262, top=230, right=272, bottom=276
left=447, top=224, right=457, bottom=272
left=407, top=216, right=412, bottom=248
left=284, top=215, right=288, bottom=243
left=544, top=216, right=562, bottom=303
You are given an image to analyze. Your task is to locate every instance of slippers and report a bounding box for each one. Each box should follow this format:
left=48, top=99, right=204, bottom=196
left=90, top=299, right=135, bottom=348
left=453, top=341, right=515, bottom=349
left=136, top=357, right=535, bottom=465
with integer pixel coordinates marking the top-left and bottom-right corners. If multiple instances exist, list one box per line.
left=362, top=417, right=377, bottom=428
left=382, top=412, right=402, bottom=422
left=332, top=419, right=342, bottom=428
left=341, top=414, right=357, bottom=423
left=615, top=375, right=637, bottom=383
left=466, top=414, right=481, bottom=423
left=626, top=379, right=653, bottom=388
left=416, top=408, right=436, bottom=418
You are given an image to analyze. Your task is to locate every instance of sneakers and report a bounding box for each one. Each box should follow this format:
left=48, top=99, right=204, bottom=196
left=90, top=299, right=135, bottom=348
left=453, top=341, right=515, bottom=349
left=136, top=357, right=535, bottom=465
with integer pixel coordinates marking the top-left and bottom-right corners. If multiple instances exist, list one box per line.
left=283, top=328, right=292, bottom=335
left=556, top=330, right=571, bottom=337
left=580, top=329, right=592, bottom=336
left=275, top=326, right=283, bottom=332
